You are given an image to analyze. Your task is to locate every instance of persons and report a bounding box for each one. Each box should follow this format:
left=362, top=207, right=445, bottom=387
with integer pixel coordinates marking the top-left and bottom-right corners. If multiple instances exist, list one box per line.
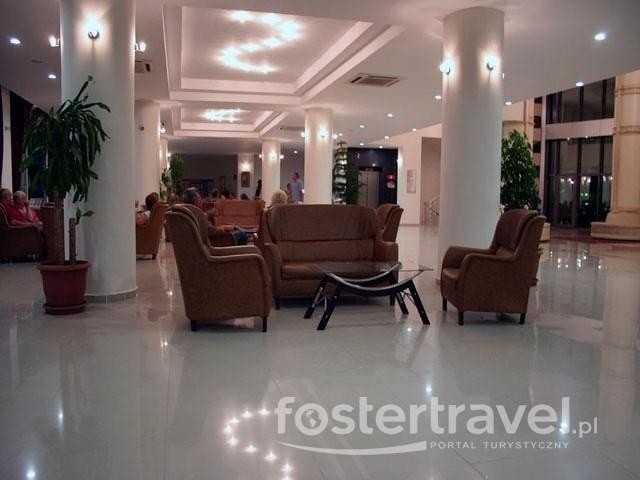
left=0, top=188, right=159, bottom=262
left=182, top=172, right=304, bottom=246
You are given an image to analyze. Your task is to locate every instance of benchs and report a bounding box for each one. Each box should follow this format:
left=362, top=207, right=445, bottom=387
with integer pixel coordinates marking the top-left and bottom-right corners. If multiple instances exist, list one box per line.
left=135, top=201, right=170, bottom=259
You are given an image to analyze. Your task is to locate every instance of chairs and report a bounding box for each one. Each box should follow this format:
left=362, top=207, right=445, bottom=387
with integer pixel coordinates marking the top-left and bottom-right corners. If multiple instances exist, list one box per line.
left=376, top=203, right=405, bottom=242
left=440, top=209, right=547, bottom=325
left=0, top=203, right=46, bottom=264
left=164, top=203, right=273, bottom=332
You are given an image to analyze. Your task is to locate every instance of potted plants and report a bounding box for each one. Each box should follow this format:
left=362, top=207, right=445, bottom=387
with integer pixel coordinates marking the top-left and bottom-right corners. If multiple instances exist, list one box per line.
left=19, top=77, right=112, bottom=314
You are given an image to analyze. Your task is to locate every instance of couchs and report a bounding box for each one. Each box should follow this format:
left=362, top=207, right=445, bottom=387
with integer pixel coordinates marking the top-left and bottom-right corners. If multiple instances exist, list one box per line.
left=206, top=197, right=267, bottom=232
left=257, top=206, right=399, bottom=308
